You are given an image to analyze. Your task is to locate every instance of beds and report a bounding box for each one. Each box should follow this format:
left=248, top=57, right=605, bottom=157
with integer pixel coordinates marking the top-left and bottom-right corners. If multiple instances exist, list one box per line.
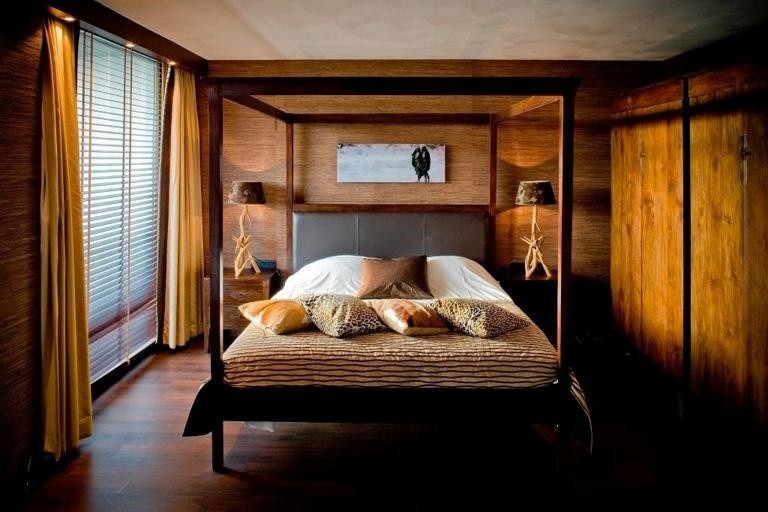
left=181, top=202, right=578, bottom=474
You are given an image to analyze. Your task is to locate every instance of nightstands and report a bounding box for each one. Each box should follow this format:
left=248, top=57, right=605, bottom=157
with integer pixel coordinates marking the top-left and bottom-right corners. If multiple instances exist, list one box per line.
left=202, top=268, right=278, bottom=353
left=509, top=266, right=557, bottom=330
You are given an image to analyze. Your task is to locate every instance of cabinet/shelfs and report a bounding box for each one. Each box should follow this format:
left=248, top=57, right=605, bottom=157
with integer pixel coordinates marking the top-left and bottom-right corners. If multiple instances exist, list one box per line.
left=607, top=75, right=687, bottom=382
left=687, top=56, right=768, bottom=418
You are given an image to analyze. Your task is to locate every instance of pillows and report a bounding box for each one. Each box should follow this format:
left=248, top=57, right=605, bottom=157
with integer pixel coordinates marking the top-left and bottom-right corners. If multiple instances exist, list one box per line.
left=426, top=297, right=530, bottom=337
left=364, top=297, right=450, bottom=335
left=237, top=299, right=312, bottom=335
left=358, top=255, right=434, bottom=300
left=299, top=294, right=386, bottom=337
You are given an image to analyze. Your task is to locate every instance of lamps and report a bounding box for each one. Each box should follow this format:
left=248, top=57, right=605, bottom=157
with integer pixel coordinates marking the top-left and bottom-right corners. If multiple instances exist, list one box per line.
left=514, top=181, right=556, bottom=277
left=227, top=180, right=266, bottom=276
